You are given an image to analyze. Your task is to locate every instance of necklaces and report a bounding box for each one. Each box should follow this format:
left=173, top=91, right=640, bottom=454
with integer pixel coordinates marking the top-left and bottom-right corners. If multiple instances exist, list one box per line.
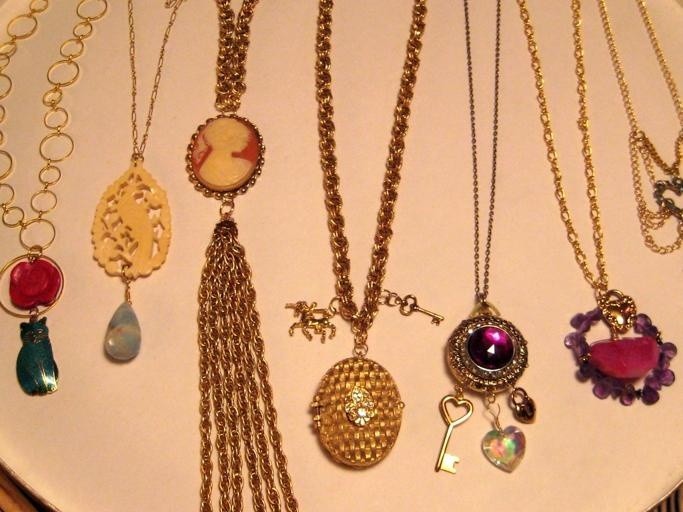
left=518, top=2, right=678, bottom=406
left=185, top=0, right=302, bottom=512
left=0, top=0, right=111, bottom=398
left=600, top=1, right=683, bottom=254
left=285, top=2, right=444, bottom=473
left=91, top=2, right=184, bottom=360
left=436, top=2, right=536, bottom=473
